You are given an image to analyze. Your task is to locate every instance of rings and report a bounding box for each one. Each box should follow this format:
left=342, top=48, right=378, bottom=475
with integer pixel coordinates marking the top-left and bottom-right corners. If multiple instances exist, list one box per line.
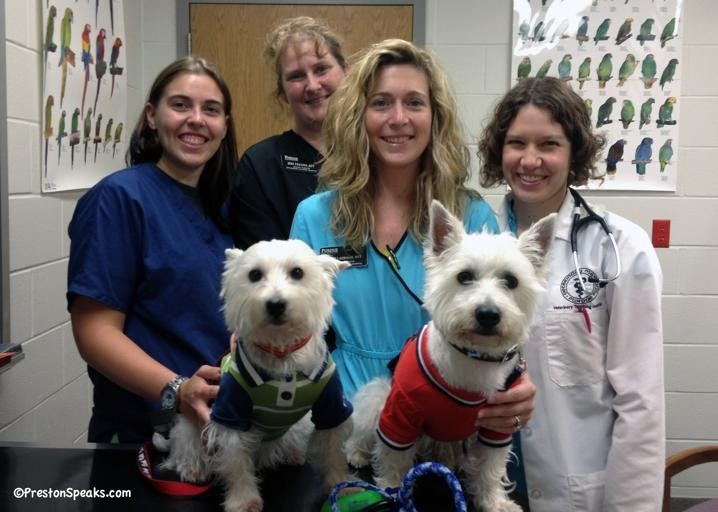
left=512, top=414, right=521, bottom=427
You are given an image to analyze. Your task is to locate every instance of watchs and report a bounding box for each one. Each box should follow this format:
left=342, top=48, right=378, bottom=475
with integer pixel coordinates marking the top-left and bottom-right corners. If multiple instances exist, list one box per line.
left=158, top=372, right=191, bottom=421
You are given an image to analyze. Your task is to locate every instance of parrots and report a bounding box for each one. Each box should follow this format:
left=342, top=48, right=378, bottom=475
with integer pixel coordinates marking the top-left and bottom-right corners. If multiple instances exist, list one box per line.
left=42, top=1, right=124, bottom=174
left=512, top=0, right=682, bottom=177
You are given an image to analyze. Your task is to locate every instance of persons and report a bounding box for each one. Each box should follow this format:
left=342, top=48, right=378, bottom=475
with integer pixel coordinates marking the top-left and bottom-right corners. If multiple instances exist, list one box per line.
left=224, top=14, right=349, bottom=250
left=65, top=54, right=240, bottom=448
left=288, top=39, right=537, bottom=437
left=476, top=74, right=667, bottom=512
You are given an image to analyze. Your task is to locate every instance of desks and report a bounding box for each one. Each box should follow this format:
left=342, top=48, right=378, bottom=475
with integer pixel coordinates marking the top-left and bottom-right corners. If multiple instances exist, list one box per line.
left=0, top=433, right=530, bottom=511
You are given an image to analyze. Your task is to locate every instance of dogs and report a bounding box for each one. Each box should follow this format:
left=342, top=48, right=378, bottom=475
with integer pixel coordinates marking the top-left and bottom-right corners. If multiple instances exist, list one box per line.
left=148, top=236, right=355, bottom=512
left=343, top=193, right=559, bottom=512
